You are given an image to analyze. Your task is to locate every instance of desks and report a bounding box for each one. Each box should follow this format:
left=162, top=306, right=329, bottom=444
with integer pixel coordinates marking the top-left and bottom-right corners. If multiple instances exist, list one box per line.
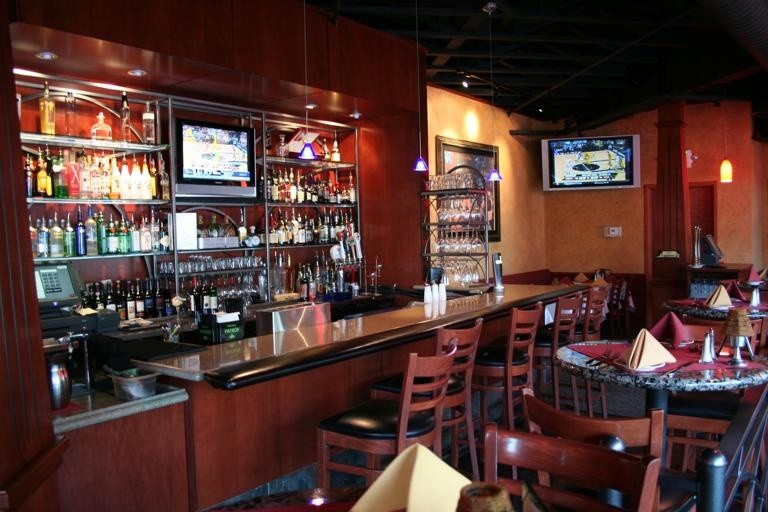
left=553, top=336, right=768, bottom=418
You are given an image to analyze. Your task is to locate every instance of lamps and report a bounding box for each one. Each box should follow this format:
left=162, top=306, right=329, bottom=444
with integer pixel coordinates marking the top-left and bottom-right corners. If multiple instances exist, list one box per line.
left=717, top=101, right=732, bottom=183
left=293, top=0, right=316, bottom=162
left=481, top=0, right=503, bottom=181
left=410, top=0, right=429, bottom=173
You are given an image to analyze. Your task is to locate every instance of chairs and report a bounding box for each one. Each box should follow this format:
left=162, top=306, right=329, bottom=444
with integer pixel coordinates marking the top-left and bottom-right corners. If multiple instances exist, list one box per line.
left=481, top=421, right=661, bottom=511
left=661, top=410, right=760, bottom=511
left=520, top=386, right=697, bottom=511
left=657, top=263, right=767, bottom=352
left=451, top=301, right=543, bottom=479
left=316, top=336, right=458, bottom=488
left=370, top=318, right=482, bottom=483
left=513, top=274, right=633, bottom=419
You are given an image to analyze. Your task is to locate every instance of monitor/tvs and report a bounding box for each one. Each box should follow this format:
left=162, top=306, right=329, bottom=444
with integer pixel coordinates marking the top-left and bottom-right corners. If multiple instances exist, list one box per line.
left=541, top=134, right=641, bottom=192
left=704, top=234, right=723, bottom=258
left=171, top=116, right=256, bottom=198
left=33, top=262, right=82, bottom=319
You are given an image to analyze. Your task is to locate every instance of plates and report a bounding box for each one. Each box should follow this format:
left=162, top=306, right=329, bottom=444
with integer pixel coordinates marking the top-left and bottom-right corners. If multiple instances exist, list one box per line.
left=660, top=338, right=696, bottom=350
left=709, top=305, right=733, bottom=311
left=613, top=356, right=665, bottom=372
left=745, top=281, right=760, bottom=286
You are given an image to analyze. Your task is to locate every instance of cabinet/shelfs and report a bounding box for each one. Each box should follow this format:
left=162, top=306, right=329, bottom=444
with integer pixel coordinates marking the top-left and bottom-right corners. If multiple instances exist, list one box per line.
left=174, top=200, right=270, bottom=322
left=263, top=109, right=363, bottom=300
left=14, top=71, right=176, bottom=336
left=420, top=165, right=489, bottom=290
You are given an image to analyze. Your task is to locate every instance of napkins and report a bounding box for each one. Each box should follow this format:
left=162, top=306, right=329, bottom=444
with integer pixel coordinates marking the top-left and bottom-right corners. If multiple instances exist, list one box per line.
left=616, top=328, right=677, bottom=370
left=349, top=442, right=472, bottom=512
left=649, top=311, right=692, bottom=347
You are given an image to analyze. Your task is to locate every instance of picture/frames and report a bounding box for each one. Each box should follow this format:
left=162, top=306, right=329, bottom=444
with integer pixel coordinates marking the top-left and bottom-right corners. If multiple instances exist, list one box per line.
left=435, top=135, right=500, bottom=244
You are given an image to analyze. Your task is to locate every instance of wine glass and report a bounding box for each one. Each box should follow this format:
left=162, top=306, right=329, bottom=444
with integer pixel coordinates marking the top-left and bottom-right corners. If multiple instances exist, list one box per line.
left=427, top=172, right=473, bottom=190
left=435, top=196, right=483, bottom=286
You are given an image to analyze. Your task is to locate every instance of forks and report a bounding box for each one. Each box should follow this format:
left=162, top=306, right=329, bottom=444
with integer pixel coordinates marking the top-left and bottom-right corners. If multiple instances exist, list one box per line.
left=584, top=346, right=621, bottom=370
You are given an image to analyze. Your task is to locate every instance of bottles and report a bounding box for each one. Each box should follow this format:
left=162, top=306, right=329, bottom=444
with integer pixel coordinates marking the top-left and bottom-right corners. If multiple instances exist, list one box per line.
left=689, top=279, right=718, bottom=298
left=196, top=208, right=357, bottom=247
left=423, top=283, right=447, bottom=304
left=423, top=304, right=446, bottom=318
left=38, top=81, right=155, bottom=145
left=29, top=204, right=169, bottom=257
left=698, top=326, right=717, bottom=364
left=750, top=288, right=760, bottom=306
left=25, top=146, right=170, bottom=199
left=259, top=166, right=355, bottom=204
left=264, top=130, right=340, bottom=162
left=82, top=254, right=268, bottom=324
left=271, top=246, right=363, bottom=302
